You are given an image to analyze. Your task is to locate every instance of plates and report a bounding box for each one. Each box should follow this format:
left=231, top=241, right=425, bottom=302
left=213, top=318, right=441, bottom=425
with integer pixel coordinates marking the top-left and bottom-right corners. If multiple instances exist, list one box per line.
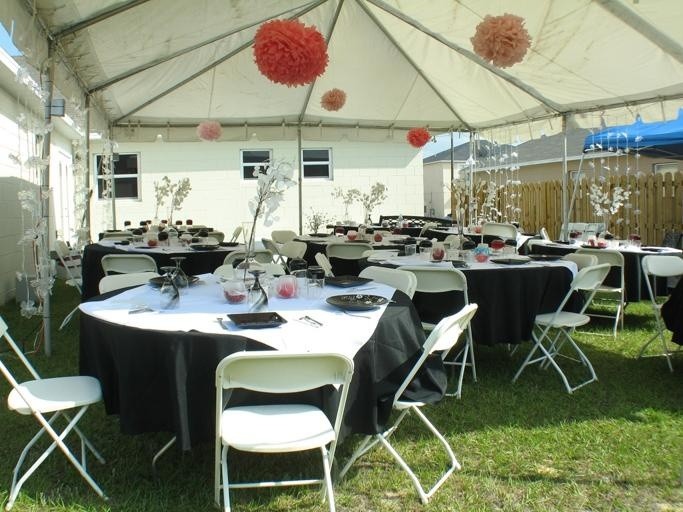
left=190, top=245, right=217, bottom=250
left=490, top=259, right=530, bottom=264
left=326, top=294, right=386, bottom=311
left=325, top=275, right=373, bottom=288
left=148, top=277, right=200, bottom=288
left=220, top=242, right=238, bottom=247
left=228, top=313, right=287, bottom=328
left=530, top=254, right=564, bottom=261
left=551, top=238, right=666, bottom=252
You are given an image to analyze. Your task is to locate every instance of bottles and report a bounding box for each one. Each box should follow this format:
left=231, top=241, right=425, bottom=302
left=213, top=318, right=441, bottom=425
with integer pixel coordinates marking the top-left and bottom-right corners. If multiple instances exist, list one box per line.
left=402, top=239, right=517, bottom=262
left=290, top=258, right=308, bottom=292
left=367, top=214, right=372, bottom=225
left=305, top=268, right=325, bottom=298
left=383, top=214, right=426, bottom=229
left=124, top=218, right=193, bottom=253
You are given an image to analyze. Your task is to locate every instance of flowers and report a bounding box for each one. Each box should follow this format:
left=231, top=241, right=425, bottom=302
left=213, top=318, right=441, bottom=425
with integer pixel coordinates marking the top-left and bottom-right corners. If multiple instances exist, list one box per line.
left=253, top=16, right=327, bottom=88
left=469, top=10, right=532, bottom=69
left=303, top=183, right=391, bottom=241
left=588, top=184, right=632, bottom=221
left=194, top=119, right=221, bottom=144
left=406, top=127, right=433, bottom=147
left=441, top=177, right=508, bottom=219
left=152, top=175, right=192, bottom=222
left=242, top=159, right=298, bottom=283
left=319, top=88, right=347, bottom=112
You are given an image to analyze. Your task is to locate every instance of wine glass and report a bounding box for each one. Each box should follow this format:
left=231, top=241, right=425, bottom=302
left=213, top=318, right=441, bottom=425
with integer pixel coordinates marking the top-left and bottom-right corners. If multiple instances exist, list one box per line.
left=171, top=257, right=189, bottom=294
left=159, top=266, right=180, bottom=310
left=247, top=269, right=268, bottom=312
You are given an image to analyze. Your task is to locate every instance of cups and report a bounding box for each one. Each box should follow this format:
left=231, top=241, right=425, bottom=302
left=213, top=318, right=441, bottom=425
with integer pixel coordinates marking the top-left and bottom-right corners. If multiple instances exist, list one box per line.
left=225, top=279, right=246, bottom=301
left=336, top=226, right=382, bottom=245
left=276, top=275, right=298, bottom=296
